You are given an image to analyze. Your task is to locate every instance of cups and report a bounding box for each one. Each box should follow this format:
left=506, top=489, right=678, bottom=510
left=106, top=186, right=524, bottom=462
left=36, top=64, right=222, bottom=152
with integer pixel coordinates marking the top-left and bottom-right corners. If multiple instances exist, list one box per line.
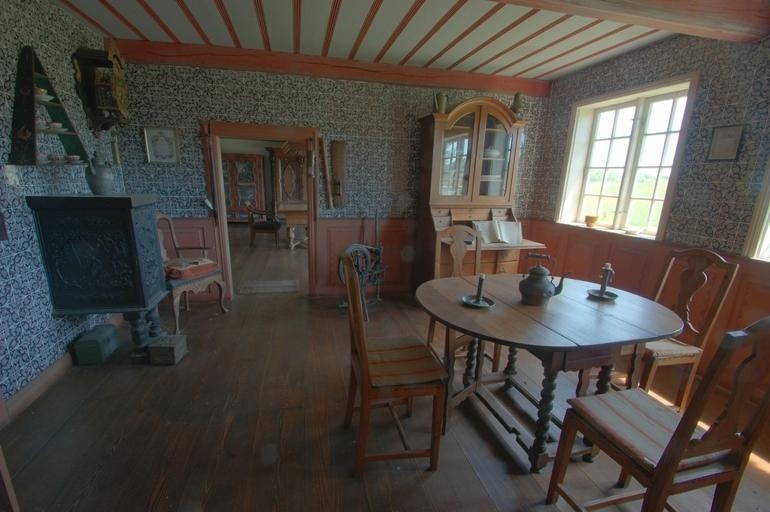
left=55, top=155, right=66, bottom=161
left=70, top=156, right=80, bottom=160
left=49, top=122, right=63, bottom=127
left=585, top=215, right=599, bottom=227
left=34, top=87, right=48, bottom=94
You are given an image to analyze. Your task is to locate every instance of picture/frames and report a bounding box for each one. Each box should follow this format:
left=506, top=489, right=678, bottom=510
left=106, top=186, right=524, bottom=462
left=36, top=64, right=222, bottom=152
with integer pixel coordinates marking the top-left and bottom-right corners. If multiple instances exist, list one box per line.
left=143, top=124, right=181, bottom=166
left=704, top=124, right=746, bottom=162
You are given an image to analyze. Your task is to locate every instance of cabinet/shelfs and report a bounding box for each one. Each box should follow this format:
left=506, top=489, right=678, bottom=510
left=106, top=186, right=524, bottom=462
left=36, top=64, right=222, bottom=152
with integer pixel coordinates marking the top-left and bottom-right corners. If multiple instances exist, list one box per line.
left=8, top=46, right=92, bottom=165
left=221, top=152, right=269, bottom=223
left=413, top=96, right=547, bottom=279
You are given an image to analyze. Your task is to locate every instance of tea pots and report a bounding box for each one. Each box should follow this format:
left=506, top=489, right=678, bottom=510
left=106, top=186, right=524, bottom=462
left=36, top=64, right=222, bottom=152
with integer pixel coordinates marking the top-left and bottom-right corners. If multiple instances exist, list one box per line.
left=85, top=150, right=115, bottom=196
left=519, top=253, right=576, bottom=307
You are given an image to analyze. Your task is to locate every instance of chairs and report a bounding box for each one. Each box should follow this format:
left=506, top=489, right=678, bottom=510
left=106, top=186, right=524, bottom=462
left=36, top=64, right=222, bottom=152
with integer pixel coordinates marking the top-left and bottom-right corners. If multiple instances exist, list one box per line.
left=152, top=211, right=230, bottom=333
left=246, top=203, right=281, bottom=249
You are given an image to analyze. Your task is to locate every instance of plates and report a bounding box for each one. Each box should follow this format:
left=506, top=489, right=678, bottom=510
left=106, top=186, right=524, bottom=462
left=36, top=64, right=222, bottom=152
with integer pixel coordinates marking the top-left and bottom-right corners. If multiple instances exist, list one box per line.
left=34, top=94, right=54, bottom=101
left=53, top=160, right=83, bottom=166
left=49, top=127, right=69, bottom=132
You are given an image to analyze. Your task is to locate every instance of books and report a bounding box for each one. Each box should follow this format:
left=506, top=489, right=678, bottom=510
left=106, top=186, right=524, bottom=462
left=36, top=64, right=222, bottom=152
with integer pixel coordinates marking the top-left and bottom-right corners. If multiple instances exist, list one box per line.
left=472, top=219, right=523, bottom=246
